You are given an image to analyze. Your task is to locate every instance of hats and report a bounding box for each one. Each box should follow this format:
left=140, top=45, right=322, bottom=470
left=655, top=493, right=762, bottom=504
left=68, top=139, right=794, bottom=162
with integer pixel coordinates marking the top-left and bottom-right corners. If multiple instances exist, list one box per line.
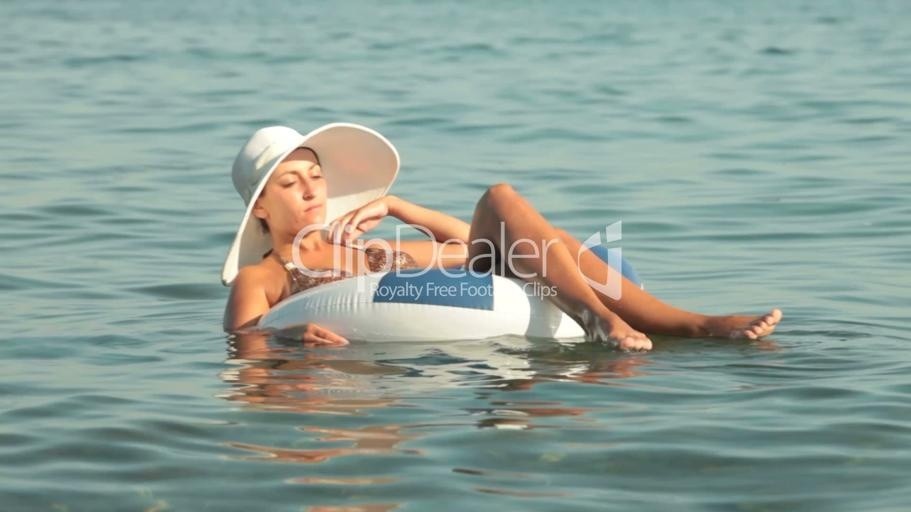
left=223, top=122, right=400, bottom=284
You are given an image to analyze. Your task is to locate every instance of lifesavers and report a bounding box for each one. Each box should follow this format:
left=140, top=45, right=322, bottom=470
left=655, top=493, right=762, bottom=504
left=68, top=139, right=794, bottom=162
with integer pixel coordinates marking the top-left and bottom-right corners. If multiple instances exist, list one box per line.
left=258, top=239, right=647, bottom=341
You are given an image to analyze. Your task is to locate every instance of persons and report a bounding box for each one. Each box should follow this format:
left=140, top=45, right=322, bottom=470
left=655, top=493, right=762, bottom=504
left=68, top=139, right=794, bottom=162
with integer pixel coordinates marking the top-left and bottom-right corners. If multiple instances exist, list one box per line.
left=224, top=122, right=782, bottom=351
